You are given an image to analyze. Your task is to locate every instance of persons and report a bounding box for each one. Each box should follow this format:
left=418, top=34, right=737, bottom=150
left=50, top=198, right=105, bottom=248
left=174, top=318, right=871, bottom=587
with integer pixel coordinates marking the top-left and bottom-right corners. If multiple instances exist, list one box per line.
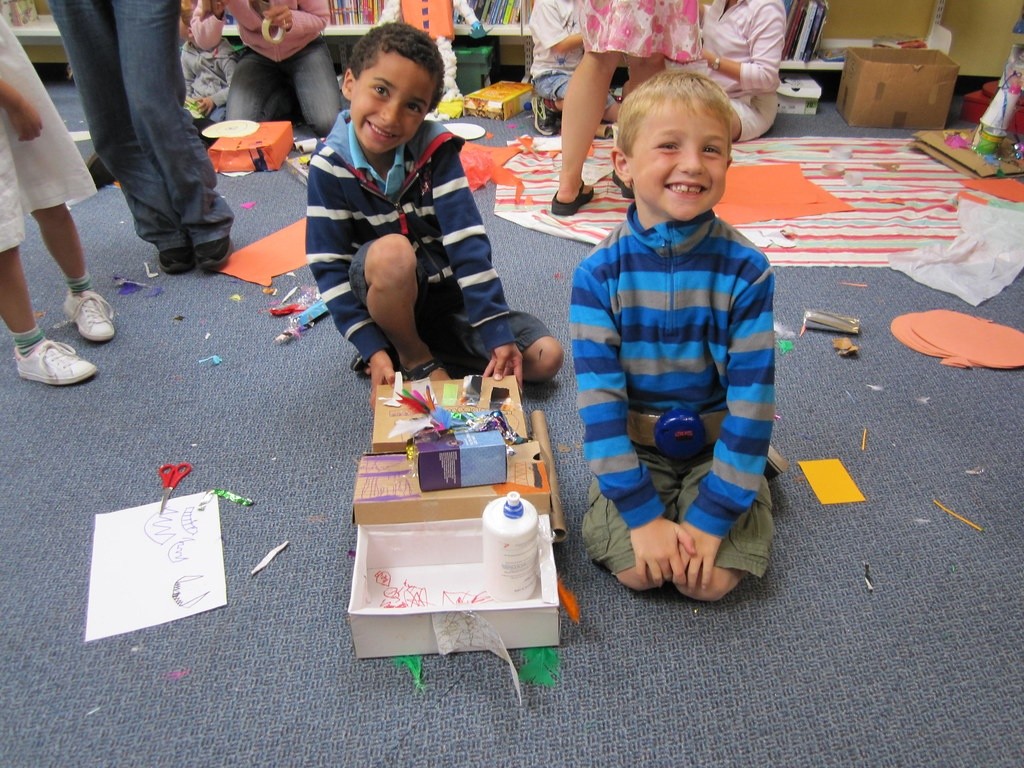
left=47, top=0, right=238, bottom=276
left=545, top=0, right=701, bottom=218
left=0, top=0, right=117, bottom=389
left=179, top=0, right=238, bottom=149
left=664, top=1, right=788, bottom=140
left=310, top=18, right=570, bottom=420
left=524, top=0, right=622, bottom=139
left=189, top=0, right=341, bottom=139
left=566, top=65, right=790, bottom=605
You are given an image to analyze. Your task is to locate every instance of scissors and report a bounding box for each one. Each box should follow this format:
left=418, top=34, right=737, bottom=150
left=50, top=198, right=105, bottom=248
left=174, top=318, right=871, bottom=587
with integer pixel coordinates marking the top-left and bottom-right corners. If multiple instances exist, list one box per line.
left=159, top=462, right=192, bottom=515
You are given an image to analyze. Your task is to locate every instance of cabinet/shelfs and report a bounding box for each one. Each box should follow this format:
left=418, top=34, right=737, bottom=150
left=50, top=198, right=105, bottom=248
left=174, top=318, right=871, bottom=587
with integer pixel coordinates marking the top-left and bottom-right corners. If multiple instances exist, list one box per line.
left=779, top=1, right=947, bottom=72
left=322, top=1, right=535, bottom=83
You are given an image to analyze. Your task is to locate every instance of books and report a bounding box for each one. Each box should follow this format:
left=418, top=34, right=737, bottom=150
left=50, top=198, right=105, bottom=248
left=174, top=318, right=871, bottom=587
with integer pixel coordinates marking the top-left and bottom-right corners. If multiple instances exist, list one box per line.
left=782, top=2, right=850, bottom=66
left=320, top=1, right=387, bottom=27
left=453, top=3, right=539, bottom=24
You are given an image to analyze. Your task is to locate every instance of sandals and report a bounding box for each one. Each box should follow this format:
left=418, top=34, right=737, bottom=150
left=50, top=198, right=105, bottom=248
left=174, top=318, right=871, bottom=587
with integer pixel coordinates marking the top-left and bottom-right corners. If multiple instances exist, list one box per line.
left=398, top=359, right=446, bottom=382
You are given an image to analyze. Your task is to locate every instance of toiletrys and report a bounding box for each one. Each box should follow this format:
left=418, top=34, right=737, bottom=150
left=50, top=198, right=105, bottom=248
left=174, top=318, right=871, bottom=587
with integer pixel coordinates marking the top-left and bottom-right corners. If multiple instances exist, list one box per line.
left=483, top=491, right=540, bottom=602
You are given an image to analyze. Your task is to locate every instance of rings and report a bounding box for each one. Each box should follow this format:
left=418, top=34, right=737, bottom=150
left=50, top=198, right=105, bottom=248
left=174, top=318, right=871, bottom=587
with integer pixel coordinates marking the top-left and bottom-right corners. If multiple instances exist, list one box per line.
left=282, top=19, right=288, bottom=25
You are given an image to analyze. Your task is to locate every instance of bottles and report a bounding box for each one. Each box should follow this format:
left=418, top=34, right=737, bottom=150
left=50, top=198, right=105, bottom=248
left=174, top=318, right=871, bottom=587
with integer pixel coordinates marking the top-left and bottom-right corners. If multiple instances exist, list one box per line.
left=479, top=489, right=539, bottom=603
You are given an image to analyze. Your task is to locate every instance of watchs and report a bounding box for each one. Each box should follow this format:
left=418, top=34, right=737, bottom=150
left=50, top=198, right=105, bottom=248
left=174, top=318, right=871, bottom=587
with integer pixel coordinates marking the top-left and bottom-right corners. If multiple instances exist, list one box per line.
left=710, top=56, right=722, bottom=70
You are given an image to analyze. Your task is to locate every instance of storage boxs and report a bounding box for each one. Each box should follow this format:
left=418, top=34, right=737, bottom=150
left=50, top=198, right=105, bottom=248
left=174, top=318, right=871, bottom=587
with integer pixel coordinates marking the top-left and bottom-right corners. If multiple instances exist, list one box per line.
left=210, top=120, right=295, bottom=172
left=450, top=43, right=498, bottom=94
left=834, top=44, right=960, bottom=131
left=776, top=73, right=821, bottom=115
left=344, top=513, right=562, bottom=660
left=461, top=80, right=535, bottom=121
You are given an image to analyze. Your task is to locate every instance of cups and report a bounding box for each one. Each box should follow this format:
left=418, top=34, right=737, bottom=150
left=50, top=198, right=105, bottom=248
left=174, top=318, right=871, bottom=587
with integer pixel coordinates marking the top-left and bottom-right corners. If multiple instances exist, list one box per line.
left=971, top=90, right=1019, bottom=154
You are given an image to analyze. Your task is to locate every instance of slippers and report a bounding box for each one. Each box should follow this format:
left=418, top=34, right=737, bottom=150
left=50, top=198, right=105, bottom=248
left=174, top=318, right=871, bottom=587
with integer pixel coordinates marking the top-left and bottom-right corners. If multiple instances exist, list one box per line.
left=612, top=170, right=635, bottom=198
left=551, top=180, right=595, bottom=215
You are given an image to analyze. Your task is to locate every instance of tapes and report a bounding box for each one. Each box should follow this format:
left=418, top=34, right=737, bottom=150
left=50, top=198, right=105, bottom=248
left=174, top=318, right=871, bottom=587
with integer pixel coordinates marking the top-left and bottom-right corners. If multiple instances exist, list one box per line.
left=262, top=18, right=286, bottom=44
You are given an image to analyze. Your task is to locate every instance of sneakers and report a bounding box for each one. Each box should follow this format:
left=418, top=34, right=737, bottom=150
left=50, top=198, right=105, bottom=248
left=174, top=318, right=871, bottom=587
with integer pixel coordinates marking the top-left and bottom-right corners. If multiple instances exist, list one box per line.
left=63, top=288, right=115, bottom=341
left=14, top=341, right=96, bottom=386
left=530, top=97, right=559, bottom=135
left=195, top=237, right=235, bottom=268
left=160, top=246, right=195, bottom=273
